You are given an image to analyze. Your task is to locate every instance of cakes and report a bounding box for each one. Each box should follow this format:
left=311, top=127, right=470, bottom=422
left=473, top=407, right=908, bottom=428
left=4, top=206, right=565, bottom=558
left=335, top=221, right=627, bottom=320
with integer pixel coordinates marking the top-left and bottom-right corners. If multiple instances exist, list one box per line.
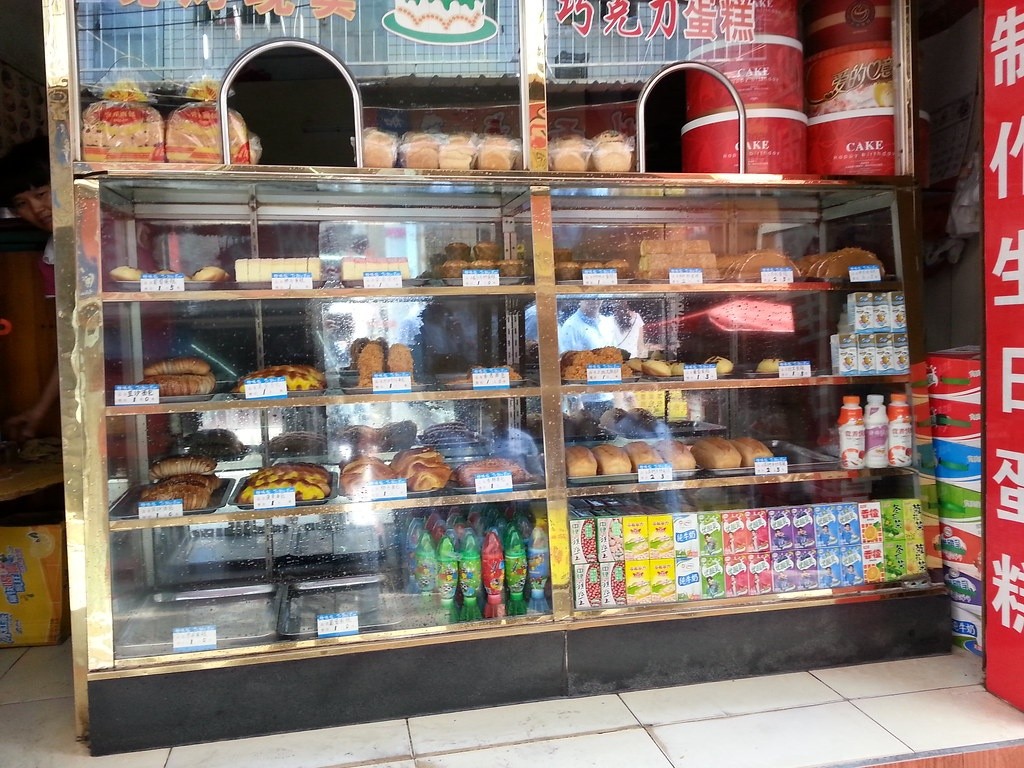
left=394, top=0, right=485, bottom=34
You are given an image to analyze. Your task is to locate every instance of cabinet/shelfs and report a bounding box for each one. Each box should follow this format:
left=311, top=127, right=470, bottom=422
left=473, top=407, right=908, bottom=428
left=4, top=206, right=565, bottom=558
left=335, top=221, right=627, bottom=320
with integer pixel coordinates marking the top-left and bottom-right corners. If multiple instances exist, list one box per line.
left=49, top=160, right=955, bottom=758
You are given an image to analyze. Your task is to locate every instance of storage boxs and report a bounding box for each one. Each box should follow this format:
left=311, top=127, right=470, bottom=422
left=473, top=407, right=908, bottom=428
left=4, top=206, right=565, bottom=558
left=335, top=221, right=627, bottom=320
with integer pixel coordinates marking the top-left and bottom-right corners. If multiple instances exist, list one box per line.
left=0, top=504, right=70, bottom=648
left=685, top=1, right=897, bottom=178
left=920, top=344, right=982, bottom=661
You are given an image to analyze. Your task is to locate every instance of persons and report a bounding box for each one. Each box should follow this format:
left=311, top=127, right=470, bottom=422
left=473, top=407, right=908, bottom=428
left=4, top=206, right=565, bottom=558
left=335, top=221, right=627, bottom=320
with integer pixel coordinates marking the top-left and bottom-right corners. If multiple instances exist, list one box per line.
left=319, top=236, right=675, bottom=521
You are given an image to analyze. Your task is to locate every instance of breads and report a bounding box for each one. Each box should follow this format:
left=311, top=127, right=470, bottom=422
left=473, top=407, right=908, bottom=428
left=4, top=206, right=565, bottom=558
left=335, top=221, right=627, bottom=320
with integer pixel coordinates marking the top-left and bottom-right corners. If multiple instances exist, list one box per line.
left=691, top=436, right=774, bottom=469
left=360, top=129, right=519, bottom=171
left=552, top=131, right=632, bottom=172
left=237, top=461, right=332, bottom=504
left=232, top=365, right=326, bottom=393
left=467, top=364, right=522, bottom=384
left=756, top=357, right=787, bottom=372
left=623, top=356, right=733, bottom=376
left=341, top=257, right=410, bottom=281
left=718, top=247, right=886, bottom=278
left=137, top=356, right=216, bottom=396
left=432, top=242, right=522, bottom=277
left=109, top=266, right=230, bottom=280
left=80, top=102, right=166, bottom=164
left=165, top=106, right=251, bottom=166
left=339, top=446, right=453, bottom=495
left=636, top=239, right=719, bottom=280
left=560, top=345, right=632, bottom=379
left=564, top=440, right=696, bottom=477
left=553, top=248, right=630, bottom=281
left=456, top=457, right=530, bottom=486
left=356, top=342, right=414, bottom=387
left=140, top=454, right=221, bottom=510
left=235, top=257, right=321, bottom=282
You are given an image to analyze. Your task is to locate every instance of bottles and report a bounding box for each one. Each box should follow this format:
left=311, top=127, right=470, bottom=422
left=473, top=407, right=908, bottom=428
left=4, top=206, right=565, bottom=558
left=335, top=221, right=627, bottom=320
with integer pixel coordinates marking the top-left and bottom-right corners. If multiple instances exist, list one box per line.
left=837, top=396, right=865, bottom=470
left=864, top=394, right=889, bottom=468
left=888, top=393, right=913, bottom=467
left=406, top=506, right=550, bottom=599
